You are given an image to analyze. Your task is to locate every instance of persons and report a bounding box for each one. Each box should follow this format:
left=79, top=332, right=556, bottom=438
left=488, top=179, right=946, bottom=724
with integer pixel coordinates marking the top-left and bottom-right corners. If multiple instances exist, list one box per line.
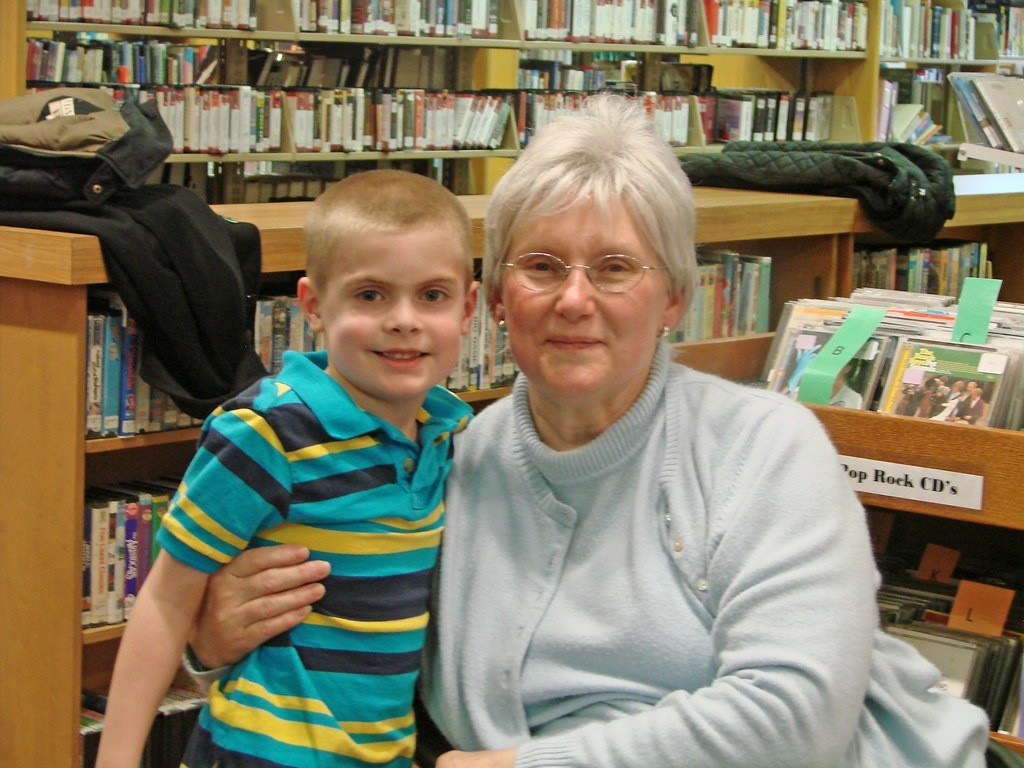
left=904, top=379, right=936, bottom=418
left=952, top=382, right=977, bottom=416
left=896, top=384, right=916, bottom=415
left=934, top=376, right=948, bottom=386
left=787, top=359, right=863, bottom=411
left=929, top=385, right=951, bottom=417
left=181, top=91, right=990, bottom=768
left=957, top=387, right=984, bottom=424
left=947, top=380, right=965, bottom=400
left=94, top=169, right=479, bottom=768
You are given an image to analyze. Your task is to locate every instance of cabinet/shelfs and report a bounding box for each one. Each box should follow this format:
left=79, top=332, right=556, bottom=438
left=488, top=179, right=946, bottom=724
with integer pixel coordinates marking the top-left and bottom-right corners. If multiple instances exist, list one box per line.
left=0, top=0, right=1024, bottom=768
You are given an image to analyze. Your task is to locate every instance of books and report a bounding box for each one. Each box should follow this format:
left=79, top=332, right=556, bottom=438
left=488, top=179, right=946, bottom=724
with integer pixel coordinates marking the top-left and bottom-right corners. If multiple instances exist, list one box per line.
left=23, top=0, right=1024, bottom=203
left=77, top=240, right=1024, bottom=768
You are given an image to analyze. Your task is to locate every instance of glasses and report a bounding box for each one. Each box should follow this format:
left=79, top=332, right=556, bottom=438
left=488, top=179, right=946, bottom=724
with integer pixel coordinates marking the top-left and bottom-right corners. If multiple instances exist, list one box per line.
left=500, top=252, right=666, bottom=294
